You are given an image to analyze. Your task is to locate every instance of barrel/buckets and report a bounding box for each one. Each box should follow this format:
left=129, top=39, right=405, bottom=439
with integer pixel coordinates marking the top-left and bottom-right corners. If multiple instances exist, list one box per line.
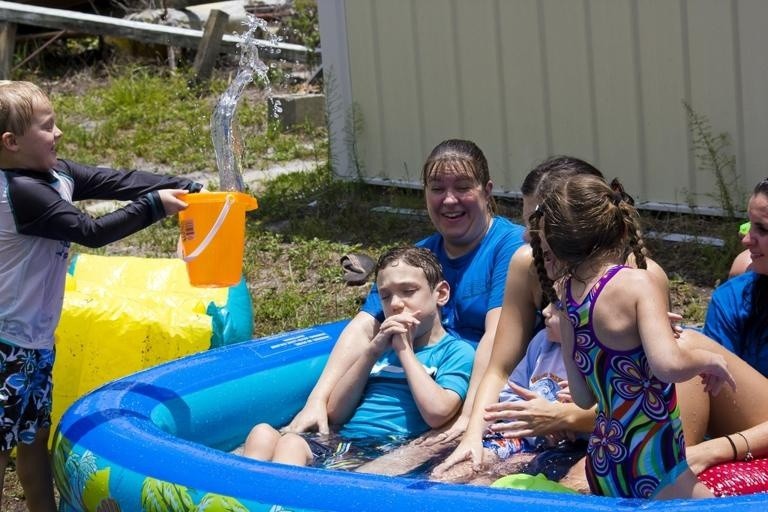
left=176, top=190, right=259, bottom=288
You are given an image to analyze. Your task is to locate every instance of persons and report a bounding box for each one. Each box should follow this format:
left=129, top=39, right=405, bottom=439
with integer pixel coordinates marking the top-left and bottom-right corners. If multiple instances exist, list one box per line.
left=725, top=220, right=755, bottom=280
left=701, top=179, right=768, bottom=375
left=1, top=78, right=209, bottom=512
left=430, top=154, right=677, bottom=490
left=232, top=242, right=473, bottom=465
left=277, top=140, right=528, bottom=441
left=530, top=172, right=739, bottom=501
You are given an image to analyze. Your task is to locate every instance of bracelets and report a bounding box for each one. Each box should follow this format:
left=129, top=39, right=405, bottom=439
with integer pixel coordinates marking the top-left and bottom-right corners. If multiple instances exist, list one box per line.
left=743, top=434, right=753, bottom=463
left=726, top=436, right=740, bottom=465
